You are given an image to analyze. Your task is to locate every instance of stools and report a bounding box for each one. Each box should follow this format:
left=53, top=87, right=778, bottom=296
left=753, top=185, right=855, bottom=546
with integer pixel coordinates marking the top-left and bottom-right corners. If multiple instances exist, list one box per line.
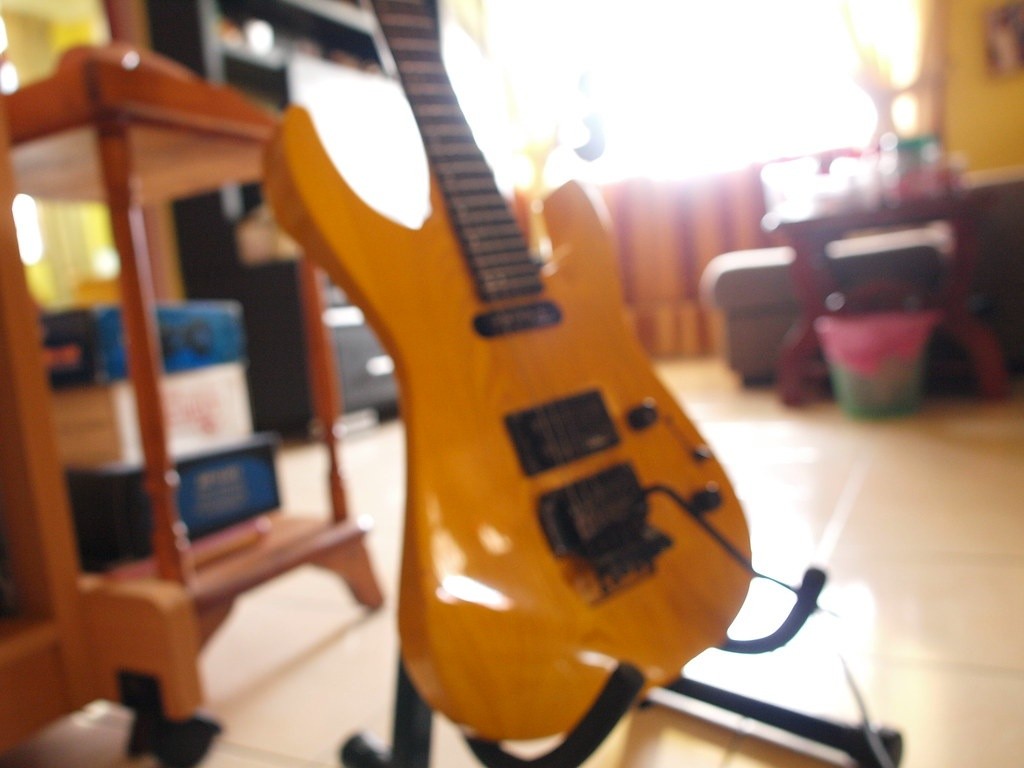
left=699, top=231, right=960, bottom=389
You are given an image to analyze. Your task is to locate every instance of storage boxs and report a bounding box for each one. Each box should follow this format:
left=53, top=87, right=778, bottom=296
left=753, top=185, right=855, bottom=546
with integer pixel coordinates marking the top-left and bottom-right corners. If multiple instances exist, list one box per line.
left=34, top=302, right=283, bottom=575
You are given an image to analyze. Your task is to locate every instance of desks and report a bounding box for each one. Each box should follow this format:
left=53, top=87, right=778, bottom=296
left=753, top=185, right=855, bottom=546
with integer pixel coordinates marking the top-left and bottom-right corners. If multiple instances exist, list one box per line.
left=760, top=165, right=1007, bottom=406
left=0, top=46, right=390, bottom=768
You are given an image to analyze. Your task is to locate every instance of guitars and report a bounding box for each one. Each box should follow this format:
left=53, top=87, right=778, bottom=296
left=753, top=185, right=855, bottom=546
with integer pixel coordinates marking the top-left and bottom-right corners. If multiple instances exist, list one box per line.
left=260, top=1, right=757, bottom=741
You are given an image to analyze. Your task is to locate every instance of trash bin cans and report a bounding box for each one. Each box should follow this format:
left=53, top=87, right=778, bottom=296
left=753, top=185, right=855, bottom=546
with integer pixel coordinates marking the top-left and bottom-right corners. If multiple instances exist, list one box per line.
left=814, top=310, right=941, bottom=419
